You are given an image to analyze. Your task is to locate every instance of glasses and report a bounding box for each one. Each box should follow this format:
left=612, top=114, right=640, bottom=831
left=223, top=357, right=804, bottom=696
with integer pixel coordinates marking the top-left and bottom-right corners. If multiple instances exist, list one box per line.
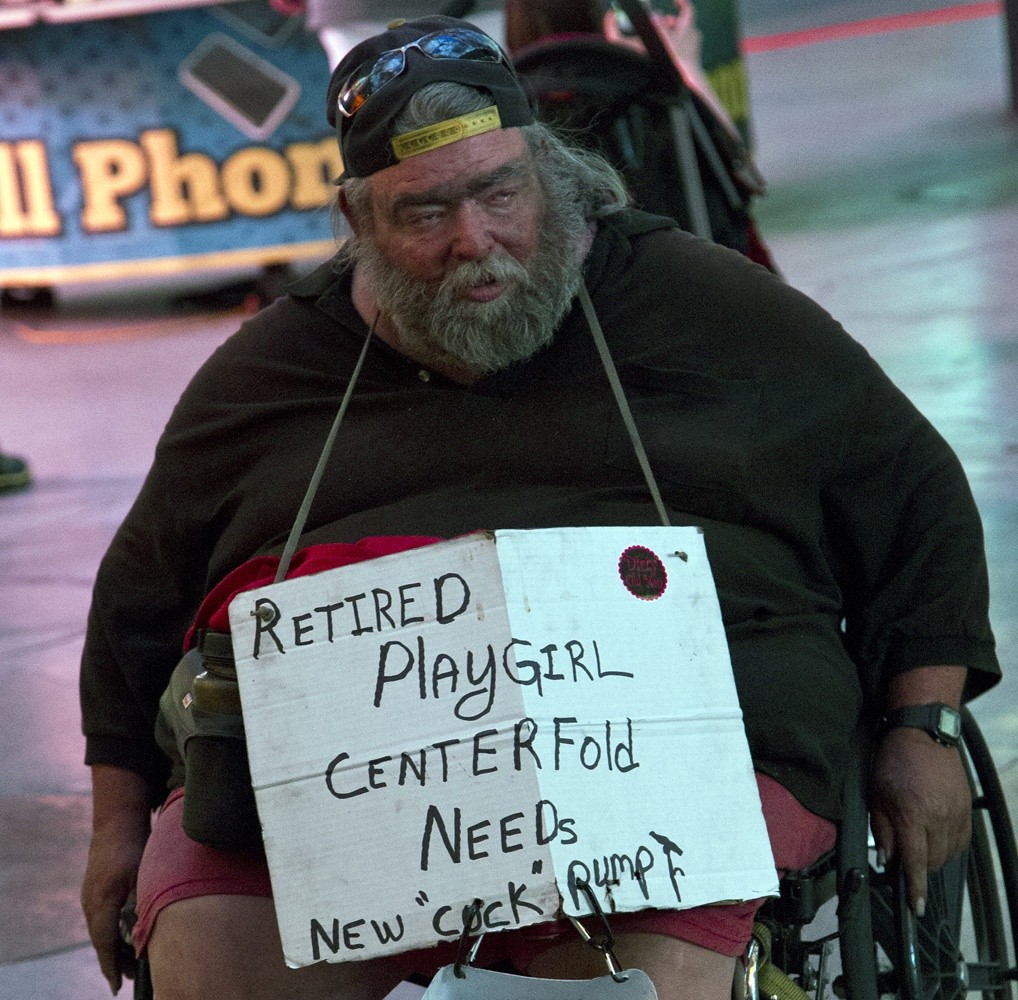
left=337, top=26, right=512, bottom=118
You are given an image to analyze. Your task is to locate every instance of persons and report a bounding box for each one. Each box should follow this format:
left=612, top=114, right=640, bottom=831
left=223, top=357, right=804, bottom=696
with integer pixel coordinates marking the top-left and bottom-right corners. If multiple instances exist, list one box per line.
left=81, top=16, right=1002, bottom=1000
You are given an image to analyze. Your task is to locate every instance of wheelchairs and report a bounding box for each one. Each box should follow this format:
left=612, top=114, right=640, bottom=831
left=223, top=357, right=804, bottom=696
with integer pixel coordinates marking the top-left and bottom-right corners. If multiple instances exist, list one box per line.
left=125, top=684, right=1018, bottom=999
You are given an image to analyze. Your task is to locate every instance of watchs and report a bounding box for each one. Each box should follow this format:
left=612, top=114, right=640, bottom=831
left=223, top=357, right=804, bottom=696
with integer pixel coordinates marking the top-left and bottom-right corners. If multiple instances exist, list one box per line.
left=878, top=700, right=962, bottom=748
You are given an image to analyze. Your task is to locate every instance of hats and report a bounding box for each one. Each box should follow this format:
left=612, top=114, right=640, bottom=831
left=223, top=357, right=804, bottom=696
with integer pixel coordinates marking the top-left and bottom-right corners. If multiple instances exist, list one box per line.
left=326, top=14, right=534, bottom=184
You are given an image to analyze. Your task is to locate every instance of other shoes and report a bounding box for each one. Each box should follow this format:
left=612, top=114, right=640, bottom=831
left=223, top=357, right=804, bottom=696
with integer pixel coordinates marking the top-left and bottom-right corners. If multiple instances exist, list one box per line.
left=1, top=454, right=29, bottom=485
